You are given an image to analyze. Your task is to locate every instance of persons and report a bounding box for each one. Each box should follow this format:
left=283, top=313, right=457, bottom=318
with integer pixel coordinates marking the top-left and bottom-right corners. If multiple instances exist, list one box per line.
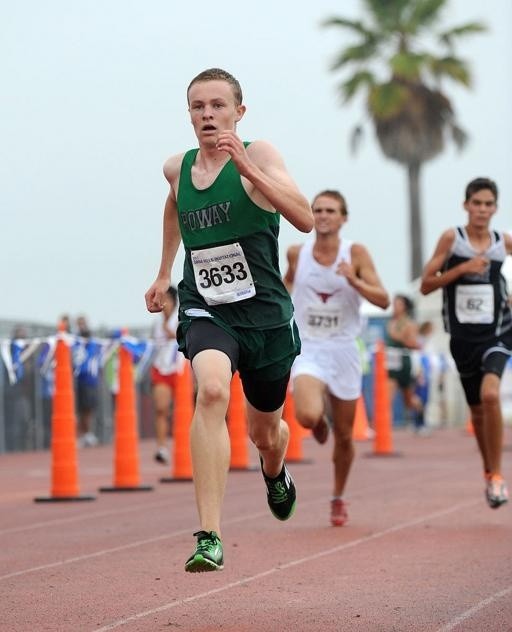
left=3, top=313, right=151, bottom=454
left=421, top=177, right=511, bottom=509
left=389, top=296, right=431, bottom=427
left=280, top=190, right=390, bottom=525
left=145, top=68, right=316, bottom=573
left=149, top=287, right=185, bottom=463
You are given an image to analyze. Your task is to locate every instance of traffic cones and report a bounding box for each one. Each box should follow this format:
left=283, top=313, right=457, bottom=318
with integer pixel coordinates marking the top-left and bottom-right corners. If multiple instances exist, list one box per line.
left=33, top=322, right=96, bottom=503
left=98, top=324, right=154, bottom=492
left=159, top=344, right=399, bottom=484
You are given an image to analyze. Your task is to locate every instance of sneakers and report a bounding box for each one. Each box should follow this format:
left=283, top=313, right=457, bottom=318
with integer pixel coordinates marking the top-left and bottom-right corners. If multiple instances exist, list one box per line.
left=485, top=476, right=508, bottom=509
left=259, top=452, right=296, bottom=520
left=186, top=531, right=224, bottom=572
left=332, top=499, right=348, bottom=527
left=312, top=416, right=328, bottom=444
